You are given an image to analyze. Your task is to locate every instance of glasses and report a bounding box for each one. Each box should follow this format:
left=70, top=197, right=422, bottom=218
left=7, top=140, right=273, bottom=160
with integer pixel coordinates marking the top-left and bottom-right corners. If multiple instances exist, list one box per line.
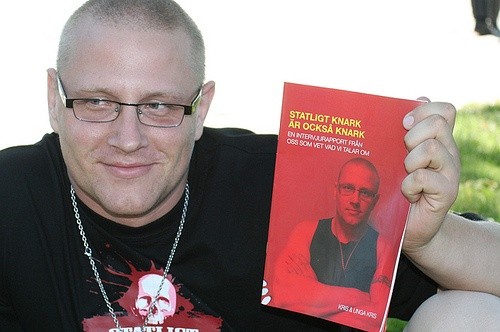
left=56, top=72, right=202, bottom=128
left=339, top=184, right=376, bottom=199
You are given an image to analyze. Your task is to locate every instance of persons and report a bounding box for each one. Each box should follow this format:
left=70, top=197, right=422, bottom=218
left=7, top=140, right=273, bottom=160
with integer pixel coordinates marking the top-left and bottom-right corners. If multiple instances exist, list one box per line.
left=0, top=0, right=500, bottom=332
left=273, top=158, right=393, bottom=332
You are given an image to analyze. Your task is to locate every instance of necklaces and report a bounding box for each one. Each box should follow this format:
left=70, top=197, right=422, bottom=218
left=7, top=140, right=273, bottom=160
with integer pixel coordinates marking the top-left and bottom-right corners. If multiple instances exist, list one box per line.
left=70, top=183, right=190, bottom=332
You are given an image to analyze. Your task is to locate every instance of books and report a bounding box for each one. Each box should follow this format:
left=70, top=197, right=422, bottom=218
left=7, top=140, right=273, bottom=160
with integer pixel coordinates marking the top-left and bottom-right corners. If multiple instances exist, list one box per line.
left=261, top=82, right=428, bottom=332
